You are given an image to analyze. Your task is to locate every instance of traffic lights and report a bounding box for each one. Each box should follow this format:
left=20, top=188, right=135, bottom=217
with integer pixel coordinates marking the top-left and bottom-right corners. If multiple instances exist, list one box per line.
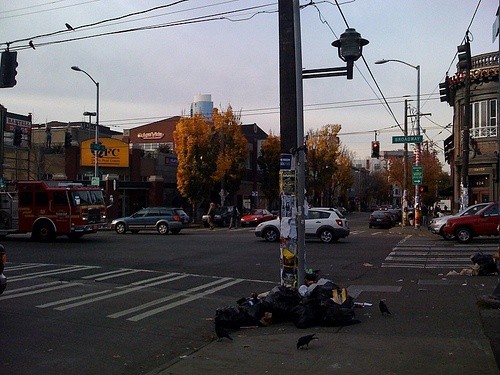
left=438, top=74, right=452, bottom=104
left=458, top=38, right=472, bottom=72
left=418, top=184, right=428, bottom=193
left=371, top=140, right=379, bottom=157
left=0, top=51, right=18, bottom=88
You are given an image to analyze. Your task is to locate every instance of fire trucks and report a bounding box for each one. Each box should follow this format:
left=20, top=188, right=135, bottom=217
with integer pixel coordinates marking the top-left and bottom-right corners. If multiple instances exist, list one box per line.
left=0, top=180, right=107, bottom=241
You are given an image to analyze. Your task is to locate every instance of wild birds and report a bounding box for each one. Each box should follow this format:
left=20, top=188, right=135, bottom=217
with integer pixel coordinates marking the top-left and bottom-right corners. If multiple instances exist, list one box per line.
left=379, top=301, right=390, bottom=315
left=297, top=334, right=319, bottom=350
left=215, top=317, right=233, bottom=341
left=251, top=292, right=258, bottom=300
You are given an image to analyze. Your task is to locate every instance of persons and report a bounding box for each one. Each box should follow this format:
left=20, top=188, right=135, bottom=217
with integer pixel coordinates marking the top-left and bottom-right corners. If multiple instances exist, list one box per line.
left=438, top=205, right=441, bottom=213
left=422, top=204, right=428, bottom=226
left=207, top=202, right=215, bottom=230
left=228, top=201, right=240, bottom=229
left=444, top=205, right=448, bottom=216
left=45, top=125, right=51, bottom=147
left=434, top=205, right=438, bottom=216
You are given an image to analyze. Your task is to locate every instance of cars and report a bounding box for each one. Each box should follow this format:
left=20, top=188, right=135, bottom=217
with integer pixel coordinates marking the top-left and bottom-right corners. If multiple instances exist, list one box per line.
left=434, top=202, right=490, bottom=239
left=338, top=207, right=347, bottom=214
left=369, top=210, right=395, bottom=228
left=387, top=208, right=401, bottom=221
left=380, top=205, right=388, bottom=210
left=241, top=208, right=273, bottom=225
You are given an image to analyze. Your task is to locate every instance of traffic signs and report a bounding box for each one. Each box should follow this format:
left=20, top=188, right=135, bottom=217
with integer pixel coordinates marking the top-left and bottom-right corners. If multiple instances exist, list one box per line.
left=412, top=166, right=422, bottom=185
left=392, top=135, right=422, bottom=142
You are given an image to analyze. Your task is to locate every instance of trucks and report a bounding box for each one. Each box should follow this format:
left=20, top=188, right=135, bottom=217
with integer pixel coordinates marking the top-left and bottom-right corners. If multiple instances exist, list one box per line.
left=430, top=199, right=451, bottom=210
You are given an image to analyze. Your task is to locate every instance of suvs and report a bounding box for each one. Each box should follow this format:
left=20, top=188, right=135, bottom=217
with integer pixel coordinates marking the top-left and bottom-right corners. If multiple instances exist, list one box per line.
left=443, top=201, right=500, bottom=243
left=111, top=206, right=189, bottom=234
left=202, top=205, right=238, bottom=226
left=255, top=207, right=350, bottom=242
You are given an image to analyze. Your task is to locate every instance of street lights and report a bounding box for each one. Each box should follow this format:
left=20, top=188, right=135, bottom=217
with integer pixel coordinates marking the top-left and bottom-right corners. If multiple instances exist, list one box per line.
left=279, top=28, right=369, bottom=286
left=71, top=66, right=99, bottom=176
left=375, top=59, right=420, bottom=231
